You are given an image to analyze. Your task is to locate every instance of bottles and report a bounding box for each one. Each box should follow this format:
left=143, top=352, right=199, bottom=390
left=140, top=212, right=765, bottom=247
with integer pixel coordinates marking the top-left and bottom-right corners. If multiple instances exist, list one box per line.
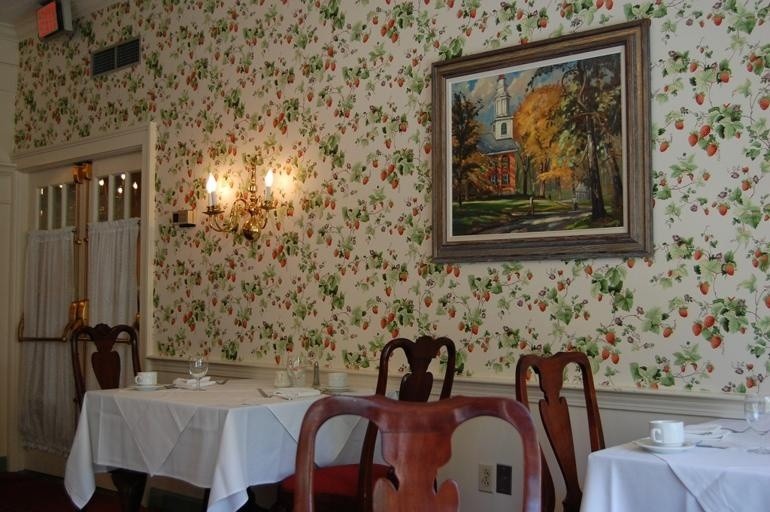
left=312, top=361, right=320, bottom=386
left=273, top=370, right=290, bottom=387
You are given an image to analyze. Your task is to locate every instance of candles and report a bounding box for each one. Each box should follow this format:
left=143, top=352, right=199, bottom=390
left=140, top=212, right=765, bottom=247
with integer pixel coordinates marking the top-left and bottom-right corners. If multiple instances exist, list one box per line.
left=264, top=167, right=274, bottom=198
left=205, top=173, right=219, bottom=208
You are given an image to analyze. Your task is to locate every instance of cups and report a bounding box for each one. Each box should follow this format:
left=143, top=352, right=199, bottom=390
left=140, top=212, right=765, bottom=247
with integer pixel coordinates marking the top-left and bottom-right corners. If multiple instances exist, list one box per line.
left=134, top=371, right=158, bottom=386
left=648, top=419, right=685, bottom=446
left=326, top=371, right=350, bottom=387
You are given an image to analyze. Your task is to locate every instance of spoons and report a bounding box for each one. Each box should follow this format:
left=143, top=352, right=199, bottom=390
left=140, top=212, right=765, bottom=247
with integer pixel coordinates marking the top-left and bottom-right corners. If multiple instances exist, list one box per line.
left=164, top=383, right=176, bottom=389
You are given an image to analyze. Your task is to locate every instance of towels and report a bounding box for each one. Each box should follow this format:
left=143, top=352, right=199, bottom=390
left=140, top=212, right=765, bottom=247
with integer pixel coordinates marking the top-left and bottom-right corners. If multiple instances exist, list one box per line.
left=687, top=415, right=770, bottom=453
left=275, top=387, right=320, bottom=398
left=173, top=376, right=216, bottom=389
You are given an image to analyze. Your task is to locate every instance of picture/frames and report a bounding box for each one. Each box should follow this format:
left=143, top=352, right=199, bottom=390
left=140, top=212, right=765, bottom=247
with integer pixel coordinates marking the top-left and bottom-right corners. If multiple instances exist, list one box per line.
left=430, top=18, right=653, bottom=264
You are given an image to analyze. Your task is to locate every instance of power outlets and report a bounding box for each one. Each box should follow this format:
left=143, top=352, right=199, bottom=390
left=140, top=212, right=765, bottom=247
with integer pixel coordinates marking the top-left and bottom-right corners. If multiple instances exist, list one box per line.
left=477, top=464, right=495, bottom=493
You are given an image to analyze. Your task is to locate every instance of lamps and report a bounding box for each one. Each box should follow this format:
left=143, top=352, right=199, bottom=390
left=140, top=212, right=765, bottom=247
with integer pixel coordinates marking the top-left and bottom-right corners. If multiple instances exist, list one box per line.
left=204, top=156, right=297, bottom=243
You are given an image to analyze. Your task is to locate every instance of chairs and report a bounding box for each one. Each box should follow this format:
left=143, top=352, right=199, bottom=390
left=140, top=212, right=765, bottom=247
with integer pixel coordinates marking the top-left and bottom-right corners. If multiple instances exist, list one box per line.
left=65, top=322, right=144, bottom=412
left=514, top=350, right=607, bottom=511
left=281, top=334, right=456, bottom=508
left=297, top=391, right=544, bottom=512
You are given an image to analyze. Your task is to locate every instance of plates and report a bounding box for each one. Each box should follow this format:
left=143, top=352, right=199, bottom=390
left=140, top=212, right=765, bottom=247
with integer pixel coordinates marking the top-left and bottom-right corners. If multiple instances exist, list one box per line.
left=633, top=439, right=695, bottom=453
left=128, top=383, right=161, bottom=390
left=324, top=383, right=354, bottom=391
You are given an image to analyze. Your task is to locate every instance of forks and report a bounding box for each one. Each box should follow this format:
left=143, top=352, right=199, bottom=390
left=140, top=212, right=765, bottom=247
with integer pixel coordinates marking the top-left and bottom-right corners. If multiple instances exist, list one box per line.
left=257, top=388, right=291, bottom=401
left=216, top=378, right=230, bottom=384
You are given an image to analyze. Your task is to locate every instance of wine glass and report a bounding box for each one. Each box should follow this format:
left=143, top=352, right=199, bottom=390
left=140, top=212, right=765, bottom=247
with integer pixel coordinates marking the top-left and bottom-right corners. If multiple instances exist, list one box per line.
left=188, top=355, right=209, bottom=391
left=744, top=393, right=770, bottom=456
